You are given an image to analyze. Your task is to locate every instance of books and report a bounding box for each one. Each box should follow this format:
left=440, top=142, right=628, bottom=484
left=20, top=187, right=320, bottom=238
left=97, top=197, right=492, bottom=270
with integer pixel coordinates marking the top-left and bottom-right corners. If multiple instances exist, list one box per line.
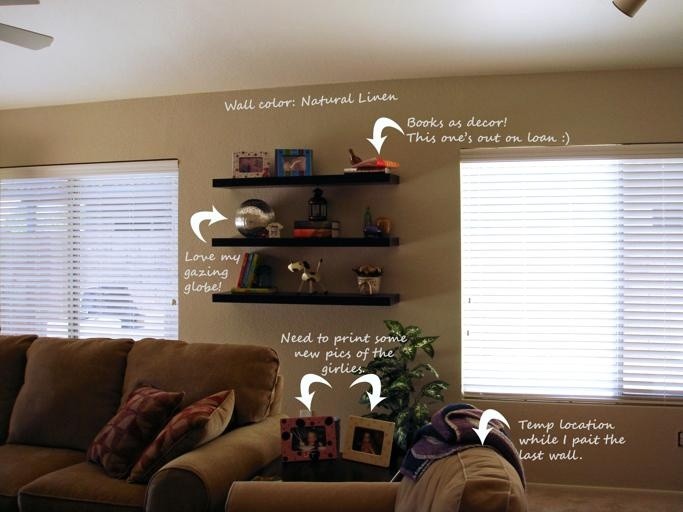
left=292, top=219, right=340, bottom=238
left=342, top=159, right=401, bottom=173
left=235, top=251, right=263, bottom=289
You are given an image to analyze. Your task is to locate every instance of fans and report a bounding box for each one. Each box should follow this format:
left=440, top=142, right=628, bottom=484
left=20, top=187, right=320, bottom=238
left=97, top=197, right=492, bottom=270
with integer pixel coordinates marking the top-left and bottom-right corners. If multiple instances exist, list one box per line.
left=0, top=0, right=54, bottom=50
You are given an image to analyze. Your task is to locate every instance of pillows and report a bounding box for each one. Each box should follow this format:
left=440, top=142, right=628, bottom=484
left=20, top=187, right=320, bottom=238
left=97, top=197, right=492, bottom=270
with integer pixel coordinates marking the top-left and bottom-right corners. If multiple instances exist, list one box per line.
left=87, top=386, right=235, bottom=480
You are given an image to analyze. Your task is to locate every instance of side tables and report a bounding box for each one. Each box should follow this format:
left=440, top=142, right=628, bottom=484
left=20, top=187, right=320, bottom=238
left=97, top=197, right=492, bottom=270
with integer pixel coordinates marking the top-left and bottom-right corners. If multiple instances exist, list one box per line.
left=249, top=455, right=402, bottom=482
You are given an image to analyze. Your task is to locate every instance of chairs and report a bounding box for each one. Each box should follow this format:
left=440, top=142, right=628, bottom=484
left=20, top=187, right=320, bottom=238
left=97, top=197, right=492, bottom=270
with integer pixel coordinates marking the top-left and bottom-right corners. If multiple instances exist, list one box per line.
left=225, top=444, right=529, bottom=512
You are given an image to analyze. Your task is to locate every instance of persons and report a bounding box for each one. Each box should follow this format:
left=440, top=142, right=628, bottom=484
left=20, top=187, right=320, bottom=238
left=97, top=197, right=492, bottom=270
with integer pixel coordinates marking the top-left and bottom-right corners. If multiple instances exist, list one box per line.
left=360, top=430, right=378, bottom=455
left=297, top=430, right=324, bottom=448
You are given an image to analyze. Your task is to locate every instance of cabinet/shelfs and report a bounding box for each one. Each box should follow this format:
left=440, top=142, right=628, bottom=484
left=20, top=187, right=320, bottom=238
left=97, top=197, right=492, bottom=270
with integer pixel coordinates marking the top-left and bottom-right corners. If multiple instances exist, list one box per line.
left=212, top=169, right=401, bottom=307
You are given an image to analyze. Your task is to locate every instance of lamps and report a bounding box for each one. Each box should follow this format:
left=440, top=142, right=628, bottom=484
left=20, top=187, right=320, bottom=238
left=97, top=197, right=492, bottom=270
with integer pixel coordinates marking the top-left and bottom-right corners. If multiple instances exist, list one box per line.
left=307, top=188, right=328, bottom=220
left=613, top=0, right=646, bottom=17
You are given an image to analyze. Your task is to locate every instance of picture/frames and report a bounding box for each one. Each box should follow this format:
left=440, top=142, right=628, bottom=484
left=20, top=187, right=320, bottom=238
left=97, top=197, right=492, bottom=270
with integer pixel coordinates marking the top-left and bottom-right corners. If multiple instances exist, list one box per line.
left=280, top=414, right=338, bottom=462
left=343, top=415, right=397, bottom=468
left=232, top=150, right=269, bottom=178
left=275, top=148, right=312, bottom=176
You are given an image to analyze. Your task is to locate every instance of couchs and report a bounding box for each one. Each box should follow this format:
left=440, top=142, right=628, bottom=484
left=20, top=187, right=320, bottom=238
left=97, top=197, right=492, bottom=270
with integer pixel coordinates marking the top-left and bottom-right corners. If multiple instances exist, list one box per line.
left=0, top=333, right=282, bottom=512
